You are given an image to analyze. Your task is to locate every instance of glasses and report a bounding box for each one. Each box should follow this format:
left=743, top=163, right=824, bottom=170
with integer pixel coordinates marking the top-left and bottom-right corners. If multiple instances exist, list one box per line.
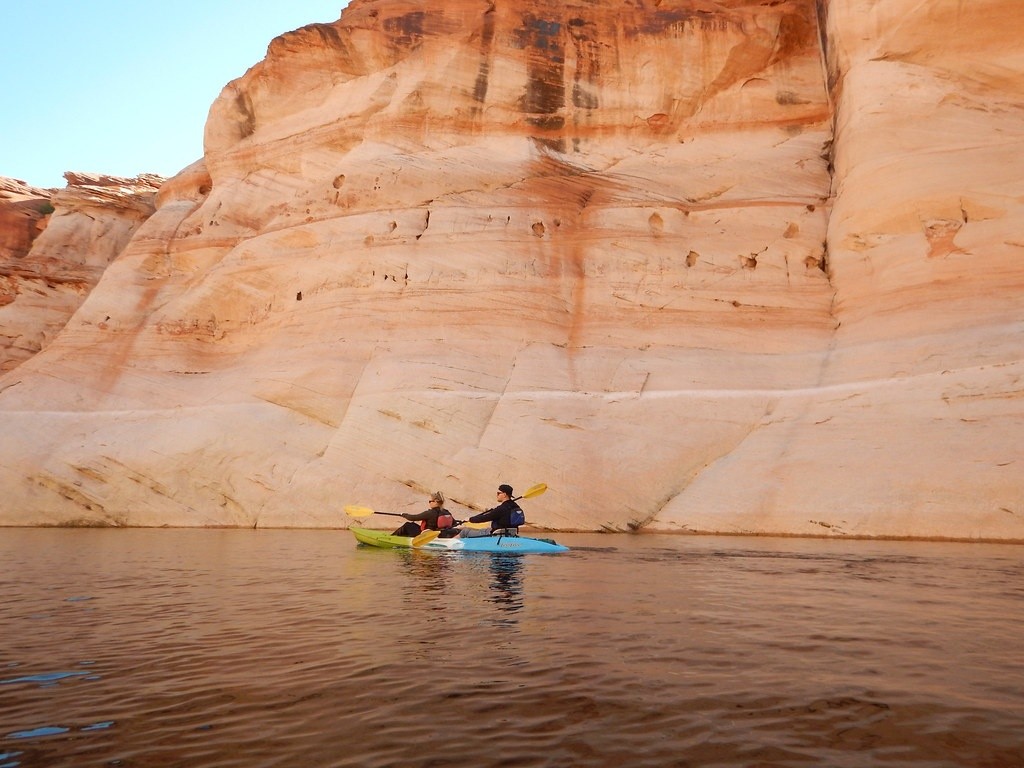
left=429, top=500, right=435, bottom=504
left=497, top=491, right=504, bottom=495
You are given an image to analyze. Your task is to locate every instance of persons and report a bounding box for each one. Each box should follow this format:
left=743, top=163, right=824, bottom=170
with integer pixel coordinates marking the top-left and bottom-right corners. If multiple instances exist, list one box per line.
left=391, top=490, right=456, bottom=538
left=452, top=485, right=525, bottom=537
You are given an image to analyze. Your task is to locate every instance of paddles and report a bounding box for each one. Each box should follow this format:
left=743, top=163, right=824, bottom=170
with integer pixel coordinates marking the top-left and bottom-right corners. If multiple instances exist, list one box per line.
left=344, top=505, right=486, bottom=529
left=413, top=484, right=547, bottom=546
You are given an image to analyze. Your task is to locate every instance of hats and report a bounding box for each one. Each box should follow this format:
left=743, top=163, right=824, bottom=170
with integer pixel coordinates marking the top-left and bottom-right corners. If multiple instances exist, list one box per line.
left=499, top=485, right=515, bottom=498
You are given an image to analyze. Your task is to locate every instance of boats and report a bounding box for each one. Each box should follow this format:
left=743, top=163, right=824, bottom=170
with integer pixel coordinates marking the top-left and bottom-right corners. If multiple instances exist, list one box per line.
left=417, top=535, right=571, bottom=555
left=348, top=526, right=425, bottom=547
